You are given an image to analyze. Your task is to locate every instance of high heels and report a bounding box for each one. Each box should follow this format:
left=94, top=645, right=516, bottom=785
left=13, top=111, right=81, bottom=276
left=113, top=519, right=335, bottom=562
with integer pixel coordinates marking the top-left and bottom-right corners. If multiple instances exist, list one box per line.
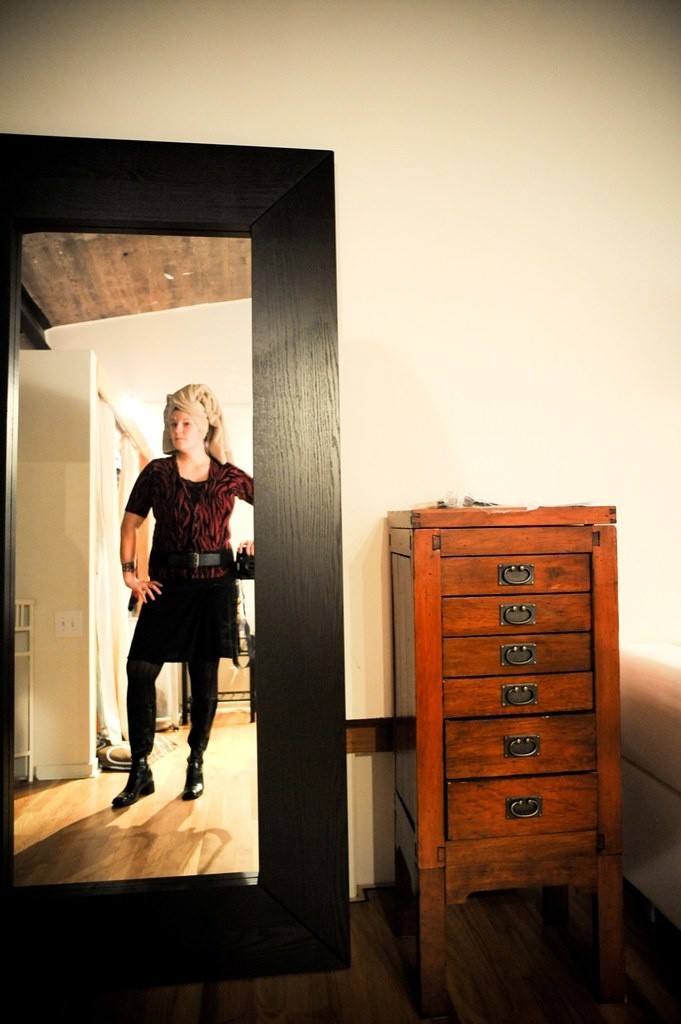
left=113, top=755, right=154, bottom=806
left=180, top=757, right=204, bottom=800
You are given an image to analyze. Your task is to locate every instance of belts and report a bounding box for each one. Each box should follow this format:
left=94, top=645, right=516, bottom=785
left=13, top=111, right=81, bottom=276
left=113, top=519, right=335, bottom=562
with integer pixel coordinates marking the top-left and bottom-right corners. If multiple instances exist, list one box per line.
left=151, top=549, right=223, bottom=568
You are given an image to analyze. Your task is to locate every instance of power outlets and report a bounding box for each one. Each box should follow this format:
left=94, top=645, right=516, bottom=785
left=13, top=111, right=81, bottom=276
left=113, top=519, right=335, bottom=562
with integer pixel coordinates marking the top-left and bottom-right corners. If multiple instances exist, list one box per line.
left=54, top=611, right=83, bottom=640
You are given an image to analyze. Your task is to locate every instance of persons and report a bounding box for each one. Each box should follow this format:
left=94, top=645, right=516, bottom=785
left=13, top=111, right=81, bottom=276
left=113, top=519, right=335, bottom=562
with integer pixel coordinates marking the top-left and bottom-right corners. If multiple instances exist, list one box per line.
left=111, top=384, right=255, bottom=810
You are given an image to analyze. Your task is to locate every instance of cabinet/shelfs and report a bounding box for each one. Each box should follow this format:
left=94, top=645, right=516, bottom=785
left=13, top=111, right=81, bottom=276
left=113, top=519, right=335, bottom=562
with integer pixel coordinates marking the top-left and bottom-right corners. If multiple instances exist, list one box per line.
left=386, top=505, right=624, bottom=1019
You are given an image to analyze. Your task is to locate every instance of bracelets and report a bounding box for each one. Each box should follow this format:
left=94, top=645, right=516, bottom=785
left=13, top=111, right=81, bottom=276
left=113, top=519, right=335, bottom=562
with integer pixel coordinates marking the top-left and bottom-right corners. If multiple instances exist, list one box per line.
left=123, top=561, right=134, bottom=573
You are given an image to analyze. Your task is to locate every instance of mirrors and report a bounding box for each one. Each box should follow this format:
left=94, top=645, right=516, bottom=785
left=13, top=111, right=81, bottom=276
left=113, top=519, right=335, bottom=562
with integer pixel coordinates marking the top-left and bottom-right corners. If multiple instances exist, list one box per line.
left=2, top=216, right=263, bottom=892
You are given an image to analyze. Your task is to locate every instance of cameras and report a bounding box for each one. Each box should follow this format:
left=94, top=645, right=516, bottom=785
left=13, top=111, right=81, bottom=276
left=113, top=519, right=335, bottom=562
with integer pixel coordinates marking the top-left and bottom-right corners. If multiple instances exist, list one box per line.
left=235, top=548, right=256, bottom=579
